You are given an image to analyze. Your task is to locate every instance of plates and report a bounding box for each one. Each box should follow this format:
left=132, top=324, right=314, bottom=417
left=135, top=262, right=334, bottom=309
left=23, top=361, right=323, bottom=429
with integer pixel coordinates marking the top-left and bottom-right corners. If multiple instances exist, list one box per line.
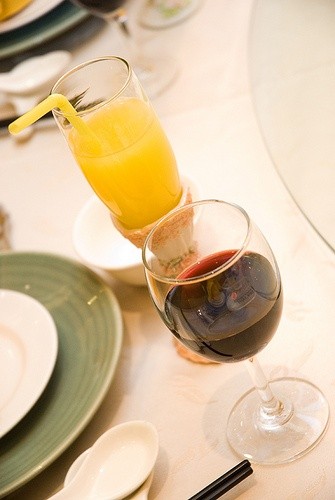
left=137, top=1, right=196, bottom=28
left=0, top=289, right=61, bottom=439
left=0, top=251, right=123, bottom=496
left=1, top=0, right=91, bottom=58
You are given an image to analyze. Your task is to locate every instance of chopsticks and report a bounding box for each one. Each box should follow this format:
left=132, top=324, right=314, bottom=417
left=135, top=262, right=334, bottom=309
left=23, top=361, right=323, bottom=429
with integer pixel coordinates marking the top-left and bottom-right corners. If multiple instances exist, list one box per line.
left=186, top=459, right=255, bottom=500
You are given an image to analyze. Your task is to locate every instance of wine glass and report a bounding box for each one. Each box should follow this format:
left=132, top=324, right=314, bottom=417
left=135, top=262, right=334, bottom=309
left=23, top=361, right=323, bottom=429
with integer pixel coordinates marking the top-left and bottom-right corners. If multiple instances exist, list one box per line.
left=76, top=0, right=172, bottom=101
left=143, top=198, right=329, bottom=467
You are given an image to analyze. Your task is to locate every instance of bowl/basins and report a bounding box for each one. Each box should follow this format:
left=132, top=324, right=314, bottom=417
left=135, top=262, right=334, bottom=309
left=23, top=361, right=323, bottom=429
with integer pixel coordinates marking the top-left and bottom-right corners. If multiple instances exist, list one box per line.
left=5, top=49, right=71, bottom=113
left=72, top=188, right=203, bottom=289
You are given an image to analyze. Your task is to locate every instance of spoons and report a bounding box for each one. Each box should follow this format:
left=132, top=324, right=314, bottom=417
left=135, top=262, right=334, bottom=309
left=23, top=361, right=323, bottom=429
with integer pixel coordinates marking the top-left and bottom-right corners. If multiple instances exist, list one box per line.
left=46, top=419, right=159, bottom=500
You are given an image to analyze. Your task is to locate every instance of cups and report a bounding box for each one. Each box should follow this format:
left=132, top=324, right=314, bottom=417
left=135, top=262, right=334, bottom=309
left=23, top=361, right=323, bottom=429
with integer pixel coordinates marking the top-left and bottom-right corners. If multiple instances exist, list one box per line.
left=50, top=57, right=221, bottom=365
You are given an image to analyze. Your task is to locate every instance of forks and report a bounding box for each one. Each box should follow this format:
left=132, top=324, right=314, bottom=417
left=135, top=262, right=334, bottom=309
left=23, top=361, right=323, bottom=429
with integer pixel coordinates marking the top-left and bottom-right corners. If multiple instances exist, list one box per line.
left=0, top=88, right=88, bottom=127
